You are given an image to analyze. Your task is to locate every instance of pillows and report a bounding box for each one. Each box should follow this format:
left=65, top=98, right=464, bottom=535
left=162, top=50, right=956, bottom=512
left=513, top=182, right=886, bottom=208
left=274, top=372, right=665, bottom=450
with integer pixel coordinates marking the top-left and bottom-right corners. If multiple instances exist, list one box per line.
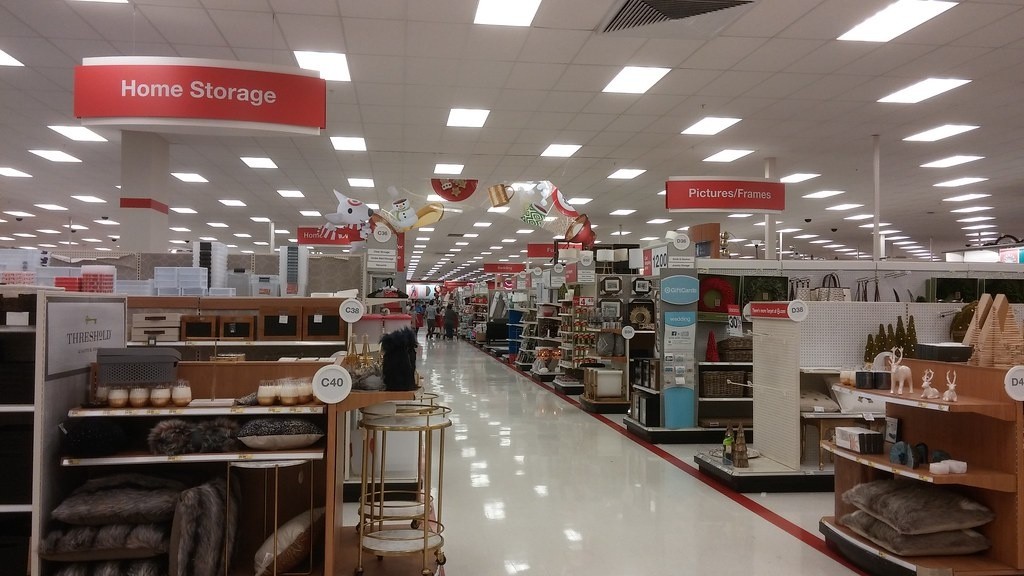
left=800, top=389, right=840, bottom=411
left=51, top=488, right=177, bottom=526
left=823, top=375, right=886, bottom=414
left=254, top=506, right=326, bottom=576
left=169, top=470, right=248, bottom=576
left=237, top=417, right=325, bottom=450
left=839, top=478, right=996, bottom=556
left=39, top=524, right=169, bottom=561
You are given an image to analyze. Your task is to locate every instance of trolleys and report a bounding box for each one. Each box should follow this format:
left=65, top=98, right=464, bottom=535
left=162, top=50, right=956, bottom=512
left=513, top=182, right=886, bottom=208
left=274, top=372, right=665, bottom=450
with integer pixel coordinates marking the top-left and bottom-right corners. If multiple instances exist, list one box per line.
left=428, top=314, right=459, bottom=339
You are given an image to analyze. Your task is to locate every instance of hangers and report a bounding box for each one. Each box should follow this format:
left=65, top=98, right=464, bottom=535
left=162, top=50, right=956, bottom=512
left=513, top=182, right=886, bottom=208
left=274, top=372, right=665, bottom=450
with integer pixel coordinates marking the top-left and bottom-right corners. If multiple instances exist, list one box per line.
left=367, top=278, right=408, bottom=298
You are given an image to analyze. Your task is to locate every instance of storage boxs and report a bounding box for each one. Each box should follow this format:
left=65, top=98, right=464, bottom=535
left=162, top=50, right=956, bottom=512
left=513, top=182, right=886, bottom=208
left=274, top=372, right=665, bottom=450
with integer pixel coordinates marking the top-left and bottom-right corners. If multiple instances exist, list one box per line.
left=506, top=324, right=523, bottom=339
left=506, top=309, right=528, bottom=324
left=302, top=306, right=347, bottom=342
left=0, top=242, right=309, bottom=344
left=354, top=313, right=383, bottom=344
left=383, top=314, right=412, bottom=334
left=96, top=348, right=181, bottom=383
left=506, top=339, right=521, bottom=354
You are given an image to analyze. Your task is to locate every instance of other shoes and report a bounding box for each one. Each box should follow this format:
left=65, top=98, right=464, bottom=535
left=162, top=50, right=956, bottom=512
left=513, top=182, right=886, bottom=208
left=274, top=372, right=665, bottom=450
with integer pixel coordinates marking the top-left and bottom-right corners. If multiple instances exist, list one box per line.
left=447, top=338, right=453, bottom=343
left=429, top=335, right=432, bottom=338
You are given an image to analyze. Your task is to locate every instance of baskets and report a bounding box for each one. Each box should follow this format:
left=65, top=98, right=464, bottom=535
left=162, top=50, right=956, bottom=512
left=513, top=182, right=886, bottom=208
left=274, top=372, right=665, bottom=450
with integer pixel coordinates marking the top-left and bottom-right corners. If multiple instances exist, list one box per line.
left=701, top=371, right=746, bottom=397
left=748, top=373, right=753, bottom=398
left=715, top=339, right=753, bottom=362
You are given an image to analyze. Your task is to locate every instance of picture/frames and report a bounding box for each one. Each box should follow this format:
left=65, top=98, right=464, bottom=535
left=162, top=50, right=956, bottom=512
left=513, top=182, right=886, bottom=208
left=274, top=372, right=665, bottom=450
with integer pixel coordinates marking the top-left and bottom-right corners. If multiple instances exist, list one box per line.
left=598, top=276, right=655, bottom=329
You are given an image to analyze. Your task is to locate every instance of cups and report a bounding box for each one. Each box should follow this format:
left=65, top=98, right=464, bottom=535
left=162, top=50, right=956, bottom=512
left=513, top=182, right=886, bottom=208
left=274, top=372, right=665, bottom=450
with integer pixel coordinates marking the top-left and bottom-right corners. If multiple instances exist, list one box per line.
left=840, top=370, right=891, bottom=389
left=257, top=377, right=324, bottom=405
left=97, top=379, right=192, bottom=407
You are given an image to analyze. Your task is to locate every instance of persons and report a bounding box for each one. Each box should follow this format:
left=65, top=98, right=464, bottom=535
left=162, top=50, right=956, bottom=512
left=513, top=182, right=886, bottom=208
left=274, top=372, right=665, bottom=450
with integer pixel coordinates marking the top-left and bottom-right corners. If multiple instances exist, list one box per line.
left=406, top=301, right=424, bottom=344
left=445, top=304, right=459, bottom=342
left=425, top=300, right=445, bottom=339
left=436, top=285, right=442, bottom=295
left=410, top=284, right=415, bottom=296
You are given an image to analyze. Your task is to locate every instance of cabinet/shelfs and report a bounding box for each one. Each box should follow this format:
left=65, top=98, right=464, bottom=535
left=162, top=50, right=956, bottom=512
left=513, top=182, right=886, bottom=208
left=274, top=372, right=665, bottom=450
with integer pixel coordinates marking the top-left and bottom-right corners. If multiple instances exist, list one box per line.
left=1, top=285, right=445, bottom=576
left=819, top=358, right=1024, bottom=576
left=694, top=301, right=1024, bottom=494
left=579, top=329, right=656, bottom=414
left=468, top=282, right=596, bottom=395
left=623, top=259, right=1024, bottom=458
left=354, top=393, right=453, bottom=576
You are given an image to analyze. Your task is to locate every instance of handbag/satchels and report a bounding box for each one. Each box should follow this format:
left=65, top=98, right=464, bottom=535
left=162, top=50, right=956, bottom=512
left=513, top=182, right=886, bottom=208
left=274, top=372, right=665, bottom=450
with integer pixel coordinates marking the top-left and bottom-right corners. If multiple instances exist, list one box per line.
left=810, top=272, right=851, bottom=301
left=416, top=314, right=423, bottom=327
